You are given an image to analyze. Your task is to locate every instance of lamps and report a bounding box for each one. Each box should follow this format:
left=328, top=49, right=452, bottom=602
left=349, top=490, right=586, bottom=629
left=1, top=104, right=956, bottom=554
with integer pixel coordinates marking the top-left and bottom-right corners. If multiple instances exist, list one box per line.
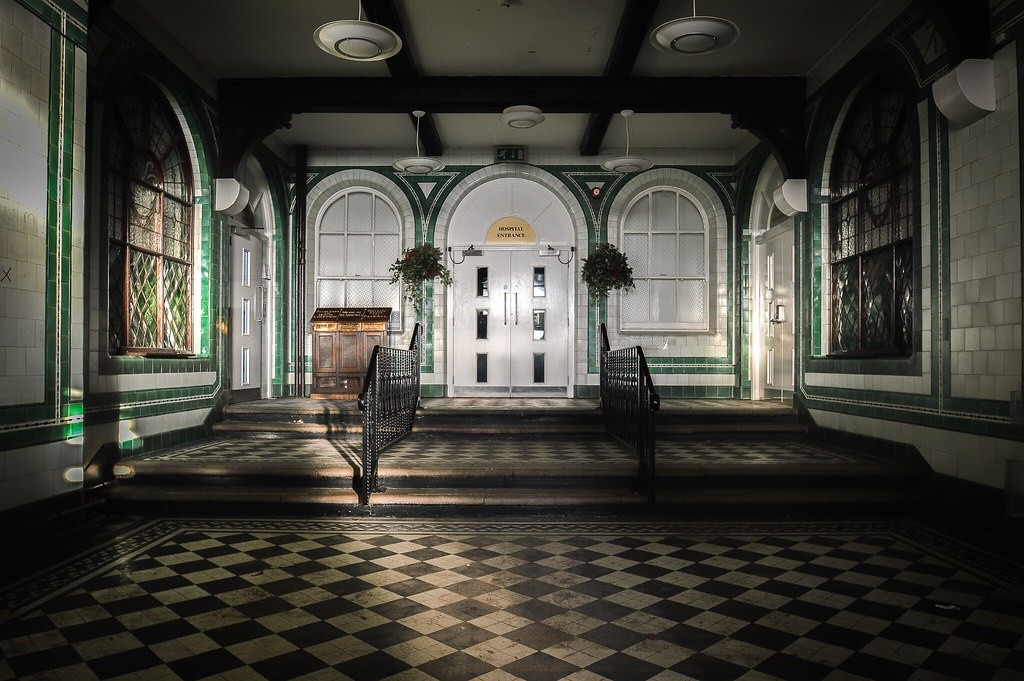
left=312, top=0, right=402, bottom=62
left=499, top=105, right=545, bottom=129
left=649, top=0, right=741, bottom=57
left=600, top=110, right=653, bottom=174
left=390, top=110, right=445, bottom=174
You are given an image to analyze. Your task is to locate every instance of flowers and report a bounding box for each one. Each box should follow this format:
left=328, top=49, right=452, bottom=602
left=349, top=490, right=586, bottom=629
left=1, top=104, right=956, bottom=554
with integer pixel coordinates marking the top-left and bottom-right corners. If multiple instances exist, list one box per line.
left=394, top=240, right=452, bottom=308
left=583, top=239, right=635, bottom=300
